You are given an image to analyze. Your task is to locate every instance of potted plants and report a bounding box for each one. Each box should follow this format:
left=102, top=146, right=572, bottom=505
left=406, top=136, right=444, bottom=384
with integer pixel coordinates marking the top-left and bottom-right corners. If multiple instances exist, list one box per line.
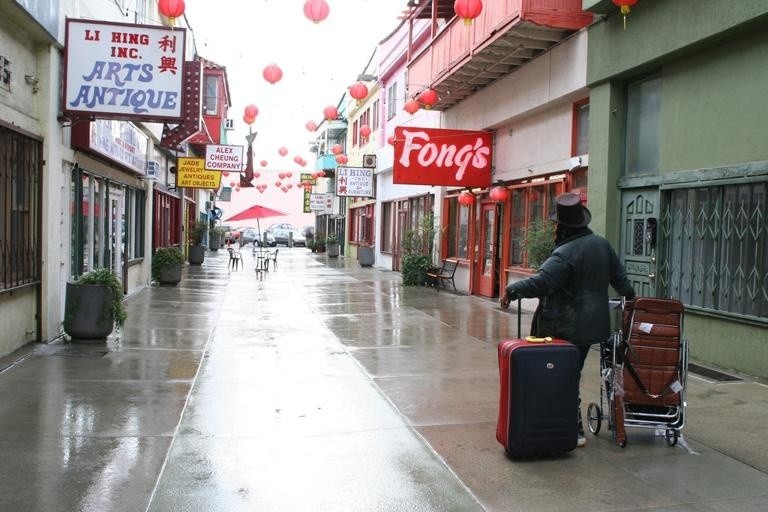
left=207, top=227, right=221, bottom=250
left=151, top=245, right=184, bottom=286
left=315, top=238, right=326, bottom=253
left=185, top=218, right=208, bottom=267
left=307, top=240, right=316, bottom=253
left=220, top=229, right=225, bottom=248
left=60, top=265, right=128, bottom=346
left=326, top=232, right=341, bottom=258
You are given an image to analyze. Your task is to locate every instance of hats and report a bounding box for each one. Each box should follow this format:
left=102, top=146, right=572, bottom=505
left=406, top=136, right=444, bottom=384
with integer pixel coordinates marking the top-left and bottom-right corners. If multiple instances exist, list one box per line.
left=549, top=193, right=591, bottom=228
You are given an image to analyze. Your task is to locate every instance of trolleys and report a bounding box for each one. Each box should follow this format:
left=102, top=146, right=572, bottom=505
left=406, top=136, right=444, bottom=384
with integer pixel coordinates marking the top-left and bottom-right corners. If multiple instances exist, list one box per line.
left=581, top=292, right=688, bottom=450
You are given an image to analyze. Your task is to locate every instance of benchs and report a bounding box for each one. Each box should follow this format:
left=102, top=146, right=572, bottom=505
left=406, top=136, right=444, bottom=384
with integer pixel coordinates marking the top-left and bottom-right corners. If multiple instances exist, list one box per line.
left=423, top=257, right=462, bottom=294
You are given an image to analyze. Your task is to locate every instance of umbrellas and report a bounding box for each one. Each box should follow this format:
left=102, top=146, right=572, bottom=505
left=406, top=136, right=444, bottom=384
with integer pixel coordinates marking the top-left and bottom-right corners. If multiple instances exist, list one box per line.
left=223, top=204, right=291, bottom=271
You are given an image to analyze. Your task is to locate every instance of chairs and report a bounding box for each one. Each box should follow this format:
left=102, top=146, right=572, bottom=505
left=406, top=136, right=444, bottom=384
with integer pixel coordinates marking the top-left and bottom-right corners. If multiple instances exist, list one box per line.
left=264, top=248, right=279, bottom=271
left=226, top=246, right=243, bottom=273
left=254, top=255, right=269, bottom=282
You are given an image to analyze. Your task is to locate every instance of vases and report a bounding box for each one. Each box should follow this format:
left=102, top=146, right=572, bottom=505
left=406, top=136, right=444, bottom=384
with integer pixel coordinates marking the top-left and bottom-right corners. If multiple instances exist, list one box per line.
left=356, top=245, right=375, bottom=267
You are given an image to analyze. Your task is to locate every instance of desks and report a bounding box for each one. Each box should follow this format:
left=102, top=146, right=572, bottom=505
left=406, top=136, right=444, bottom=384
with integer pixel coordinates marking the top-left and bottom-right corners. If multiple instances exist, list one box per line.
left=251, top=250, right=270, bottom=273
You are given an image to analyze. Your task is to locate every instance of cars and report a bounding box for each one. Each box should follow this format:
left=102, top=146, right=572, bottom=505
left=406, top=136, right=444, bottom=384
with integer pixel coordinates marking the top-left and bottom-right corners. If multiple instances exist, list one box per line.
left=272, top=229, right=306, bottom=247
left=215, top=223, right=305, bottom=244
left=237, top=228, right=278, bottom=248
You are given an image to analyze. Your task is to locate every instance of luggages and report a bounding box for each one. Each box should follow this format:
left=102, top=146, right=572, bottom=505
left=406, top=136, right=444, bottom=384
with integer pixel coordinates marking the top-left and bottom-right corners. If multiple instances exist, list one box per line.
left=495, top=292, right=579, bottom=460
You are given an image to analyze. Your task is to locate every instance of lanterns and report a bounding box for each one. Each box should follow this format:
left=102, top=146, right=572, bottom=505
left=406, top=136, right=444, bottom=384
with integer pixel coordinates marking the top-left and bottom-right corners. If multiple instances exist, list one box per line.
left=455, top=0, right=483, bottom=28
left=523, top=188, right=540, bottom=203
left=158, top=0, right=186, bottom=31
left=304, top=0, right=330, bottom=22
left=612, top=0, right=636, bottom=31
left=490, top=184, right=510, bottom=204
left=222, top=61, right=436, bottom=194
left=457, top=191, right=474, bottom=218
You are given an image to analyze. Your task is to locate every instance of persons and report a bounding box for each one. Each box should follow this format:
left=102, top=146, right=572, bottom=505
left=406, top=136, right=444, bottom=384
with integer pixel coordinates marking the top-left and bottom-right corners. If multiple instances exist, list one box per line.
left=499, top=192, right=636, bottom=447
